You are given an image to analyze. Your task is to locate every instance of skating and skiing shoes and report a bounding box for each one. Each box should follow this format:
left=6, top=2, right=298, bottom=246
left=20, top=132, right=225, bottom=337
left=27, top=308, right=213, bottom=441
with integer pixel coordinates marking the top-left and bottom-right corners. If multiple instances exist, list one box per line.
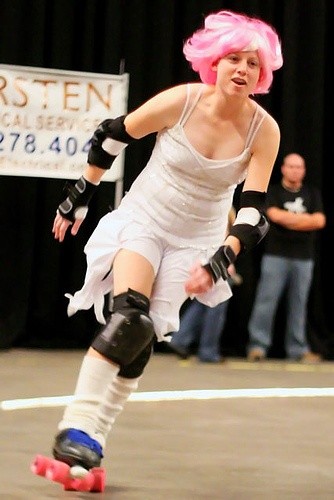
left=31, top=428, right=105, bottom=493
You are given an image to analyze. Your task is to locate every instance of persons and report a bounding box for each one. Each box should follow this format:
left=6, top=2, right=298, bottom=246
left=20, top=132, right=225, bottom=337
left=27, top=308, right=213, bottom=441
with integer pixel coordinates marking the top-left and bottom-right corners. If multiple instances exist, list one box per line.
left=31, top=9, right=283, bottom=493
left=167, top=206, right=242, bottom=364
left=247, top=153, right=325, bottom=365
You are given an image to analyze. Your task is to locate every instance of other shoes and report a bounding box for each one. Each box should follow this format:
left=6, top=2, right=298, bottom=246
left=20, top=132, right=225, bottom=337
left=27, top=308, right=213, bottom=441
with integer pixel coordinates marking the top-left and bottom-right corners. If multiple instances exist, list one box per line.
left=289, top=351, right=321, bottom=365
left=248, top=347, right=264, bottom=363
